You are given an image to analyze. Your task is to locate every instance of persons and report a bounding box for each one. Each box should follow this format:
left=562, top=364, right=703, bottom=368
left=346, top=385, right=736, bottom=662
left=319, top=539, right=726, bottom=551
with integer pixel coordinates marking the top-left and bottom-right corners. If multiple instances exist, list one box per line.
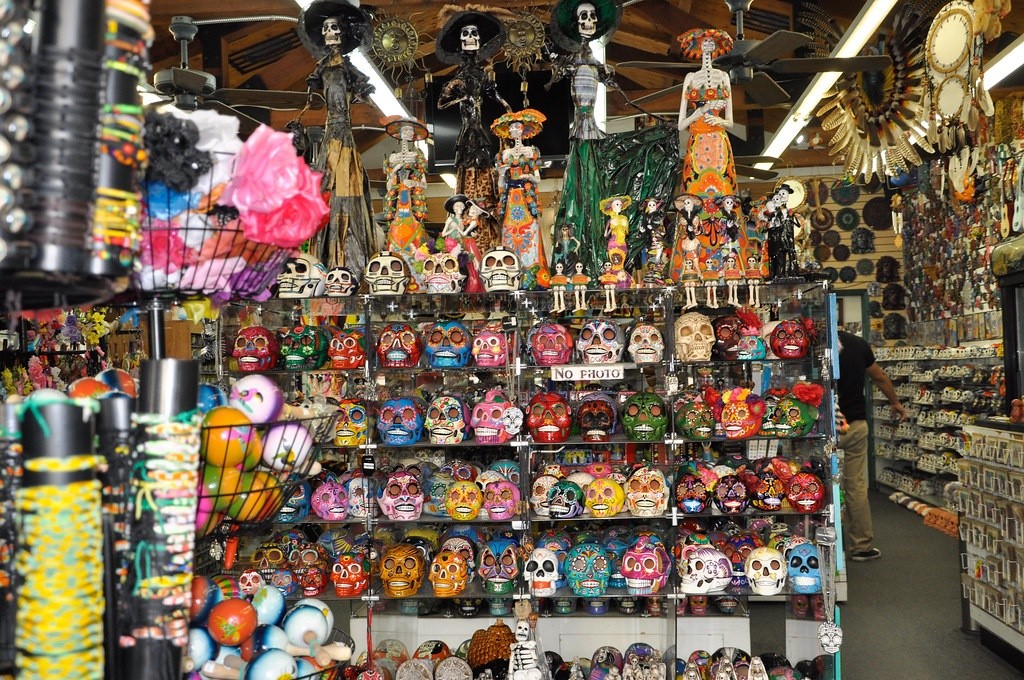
left=442, top=194, right=489, bottom=292
left=508, top=620, right=769, bottom=680
left=836, top=331, right=906, bottom=561
left=491, top=108, right=550, bottom=289
left=286, top=0, right=375, bottom=295
left=546, top=0, right=800, bottom=313
left=436, top=9, right=524, bottom=254
left=384, top=118, right=430, bottom=294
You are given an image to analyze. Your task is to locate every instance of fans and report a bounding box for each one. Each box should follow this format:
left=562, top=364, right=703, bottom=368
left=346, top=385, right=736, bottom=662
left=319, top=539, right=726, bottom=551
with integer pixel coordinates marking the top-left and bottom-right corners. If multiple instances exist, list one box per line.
left=138, top=14, right=323, bottom=136
left=606, top=1, right=895, bottom=128
left=729, top=154, right=783, bottom=181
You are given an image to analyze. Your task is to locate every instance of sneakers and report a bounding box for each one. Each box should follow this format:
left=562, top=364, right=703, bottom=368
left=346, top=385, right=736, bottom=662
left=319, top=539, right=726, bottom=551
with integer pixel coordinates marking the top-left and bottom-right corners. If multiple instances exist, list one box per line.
left=852, top=548, right=881, bottom=561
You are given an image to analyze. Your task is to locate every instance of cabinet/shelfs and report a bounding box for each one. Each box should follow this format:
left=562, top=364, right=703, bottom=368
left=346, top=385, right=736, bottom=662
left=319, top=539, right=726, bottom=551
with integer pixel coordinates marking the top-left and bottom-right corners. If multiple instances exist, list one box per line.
left=872, top=353, right=1006, bottom=506
left=194, top=277, right=843, bottom=680
